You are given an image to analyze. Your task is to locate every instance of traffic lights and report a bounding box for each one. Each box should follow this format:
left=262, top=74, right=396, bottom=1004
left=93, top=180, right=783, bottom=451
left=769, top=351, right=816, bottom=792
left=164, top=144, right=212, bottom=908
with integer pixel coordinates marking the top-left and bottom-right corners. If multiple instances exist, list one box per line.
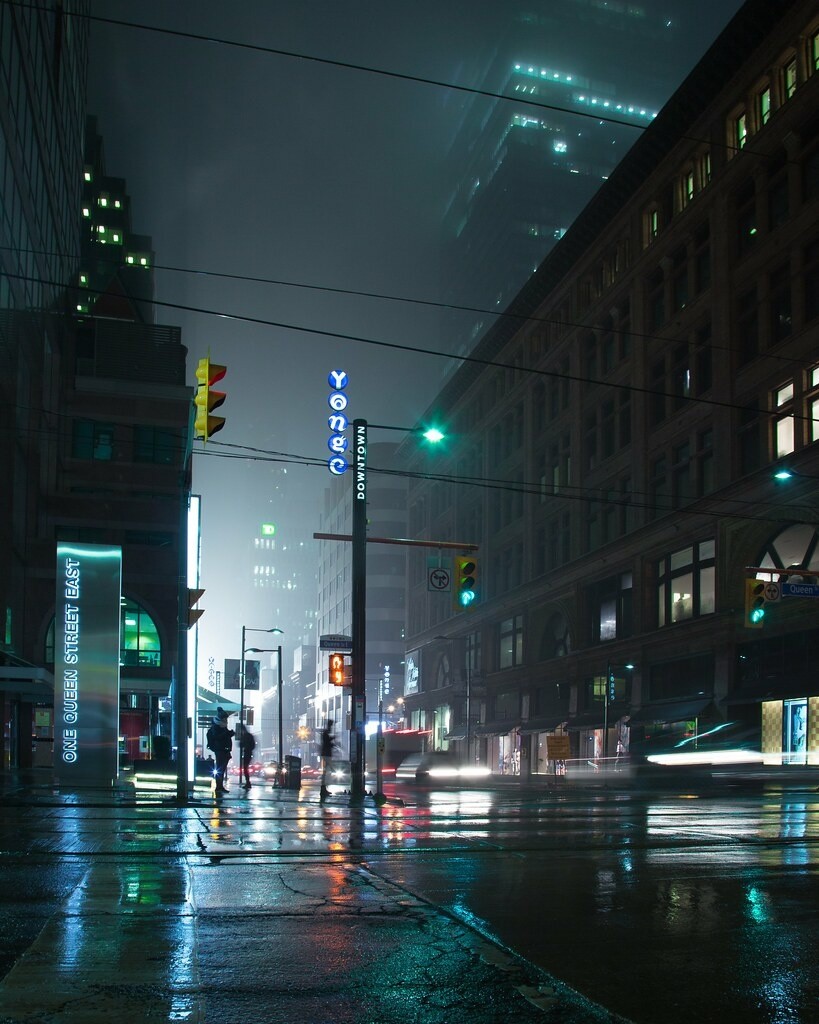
left=451, top=554, right=481, bottom=611
left=196, top=356, right=231, bottom=444
left=742, top=577, right=767, bottom=631
left=328, top=652, right=345, bottom=688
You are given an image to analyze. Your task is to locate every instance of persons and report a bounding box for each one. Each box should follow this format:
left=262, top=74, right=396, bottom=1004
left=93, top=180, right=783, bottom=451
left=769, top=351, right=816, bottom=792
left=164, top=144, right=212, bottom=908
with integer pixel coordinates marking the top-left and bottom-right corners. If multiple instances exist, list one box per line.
left=614, top=740, right=625, bottom=771
left=793, top=708, right=802, bottom=738
left=511, top=748, right=518, bottom=775
left=320, top=720, right=338, bottom=796
left=207, top=707, right=235, bottom=795
left=239, top=725, right=256, bottom=789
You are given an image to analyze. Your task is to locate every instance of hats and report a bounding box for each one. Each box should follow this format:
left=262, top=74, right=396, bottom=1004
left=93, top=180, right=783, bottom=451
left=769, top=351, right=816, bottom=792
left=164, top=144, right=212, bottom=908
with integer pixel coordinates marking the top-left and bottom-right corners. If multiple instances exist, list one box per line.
left=216, top=707, right=229, bottom=717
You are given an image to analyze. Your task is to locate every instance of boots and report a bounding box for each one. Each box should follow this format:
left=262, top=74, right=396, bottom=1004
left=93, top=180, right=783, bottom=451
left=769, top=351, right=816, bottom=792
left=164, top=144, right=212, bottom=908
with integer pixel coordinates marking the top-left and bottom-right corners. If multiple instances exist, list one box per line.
left=320, top=785, right=332, bottom=797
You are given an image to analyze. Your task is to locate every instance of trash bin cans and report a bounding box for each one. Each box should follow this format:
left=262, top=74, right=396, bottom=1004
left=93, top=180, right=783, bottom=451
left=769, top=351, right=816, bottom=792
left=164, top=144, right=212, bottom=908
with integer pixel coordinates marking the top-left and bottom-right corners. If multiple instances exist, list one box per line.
left=285, top=755, right=302, bottom=790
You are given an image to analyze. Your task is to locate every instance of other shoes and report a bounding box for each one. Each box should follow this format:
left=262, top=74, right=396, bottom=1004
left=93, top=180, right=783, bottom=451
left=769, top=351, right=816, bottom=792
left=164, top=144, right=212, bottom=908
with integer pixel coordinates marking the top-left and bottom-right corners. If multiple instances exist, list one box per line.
left=215, top=786, right=229, bottom=793
left=242, top=784, right=250, bottom=788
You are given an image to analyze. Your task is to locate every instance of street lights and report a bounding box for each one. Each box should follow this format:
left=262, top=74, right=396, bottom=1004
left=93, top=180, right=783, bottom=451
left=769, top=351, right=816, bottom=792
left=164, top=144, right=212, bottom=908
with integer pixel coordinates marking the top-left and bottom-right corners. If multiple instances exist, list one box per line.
left=434, top=634, right=472, bottom=764
left=351, top=415, right=445, bottom=800
left=246, top=645, right=286, bottom=770
left=239, top=626, right=284, bottom=788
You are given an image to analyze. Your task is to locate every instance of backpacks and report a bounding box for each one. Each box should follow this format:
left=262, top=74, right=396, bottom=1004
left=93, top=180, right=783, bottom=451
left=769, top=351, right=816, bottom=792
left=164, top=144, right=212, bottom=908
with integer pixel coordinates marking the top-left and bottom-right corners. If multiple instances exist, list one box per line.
left=206, top=728, right=215, bottom=752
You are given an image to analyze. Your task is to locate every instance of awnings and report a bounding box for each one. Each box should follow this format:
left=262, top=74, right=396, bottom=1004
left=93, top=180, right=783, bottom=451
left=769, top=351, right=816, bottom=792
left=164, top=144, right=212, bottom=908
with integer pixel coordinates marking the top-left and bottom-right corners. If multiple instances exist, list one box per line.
left=475, top=721, right=520, bottom=738
left=199, top=702, right=242, bottom=728
left=625, top=701, right=715, bottom=727
left=719, top=675, right=819, bottom=705
left=519, top=718, right=565, bottom=734
left=444, top=726, right=470, bottom=741
left=563, top=713, right=626, bottom=732
